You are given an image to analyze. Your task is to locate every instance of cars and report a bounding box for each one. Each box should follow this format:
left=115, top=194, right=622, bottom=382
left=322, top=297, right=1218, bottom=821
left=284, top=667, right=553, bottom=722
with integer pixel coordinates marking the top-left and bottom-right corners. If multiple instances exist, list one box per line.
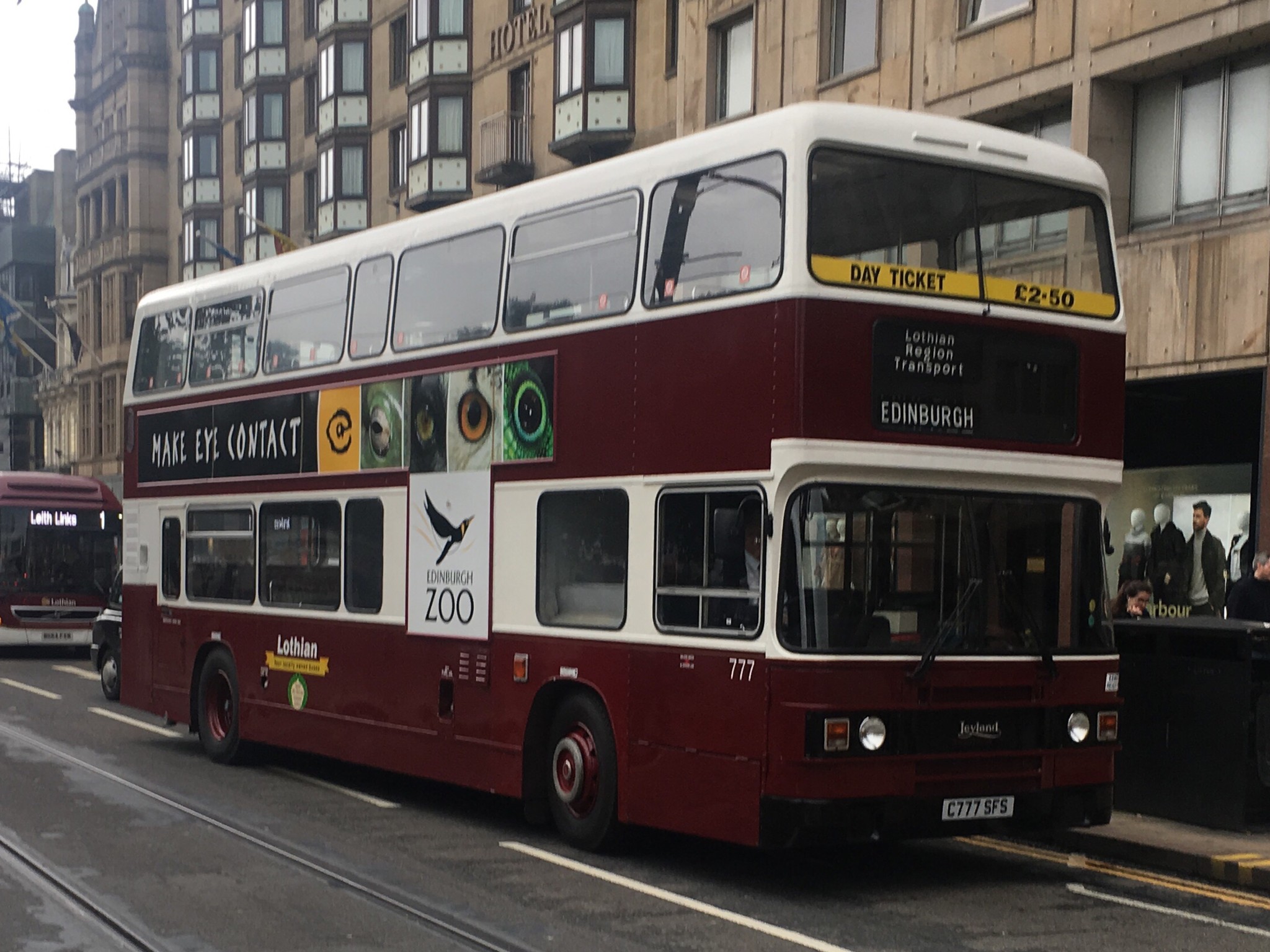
left=89, top=560, right=124, bottom=702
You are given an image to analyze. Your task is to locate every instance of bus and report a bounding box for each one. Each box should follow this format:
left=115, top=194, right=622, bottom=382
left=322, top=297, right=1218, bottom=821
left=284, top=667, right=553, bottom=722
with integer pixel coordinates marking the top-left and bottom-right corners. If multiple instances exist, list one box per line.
left=1, top=470, right=122, bottom=648
left=121, top=106, right=1125, bottom=853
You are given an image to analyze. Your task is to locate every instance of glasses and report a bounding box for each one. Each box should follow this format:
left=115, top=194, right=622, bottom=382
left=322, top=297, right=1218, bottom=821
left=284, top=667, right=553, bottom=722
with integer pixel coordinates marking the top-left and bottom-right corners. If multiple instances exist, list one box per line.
left=1134, top=595, right=1150, bottom=604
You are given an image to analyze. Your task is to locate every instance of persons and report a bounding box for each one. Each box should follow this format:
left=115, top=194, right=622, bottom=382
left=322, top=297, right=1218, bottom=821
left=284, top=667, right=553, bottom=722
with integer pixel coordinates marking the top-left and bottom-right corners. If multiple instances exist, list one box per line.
left=1227, top=551, right=1270, bottom=623
left=1186, top=501, right=1226, bottom=618
left=1111, top=579, right=1154, bottom=620
left=708, top=501, right=787, bottom=632
left=1116, top=507, right=1152, bottom=594
left=1227, top=508, right=1251, bottom=611
left=1144, top=504, right=1186, bottom=604
left=813, top=518, right=845, bottom=590
